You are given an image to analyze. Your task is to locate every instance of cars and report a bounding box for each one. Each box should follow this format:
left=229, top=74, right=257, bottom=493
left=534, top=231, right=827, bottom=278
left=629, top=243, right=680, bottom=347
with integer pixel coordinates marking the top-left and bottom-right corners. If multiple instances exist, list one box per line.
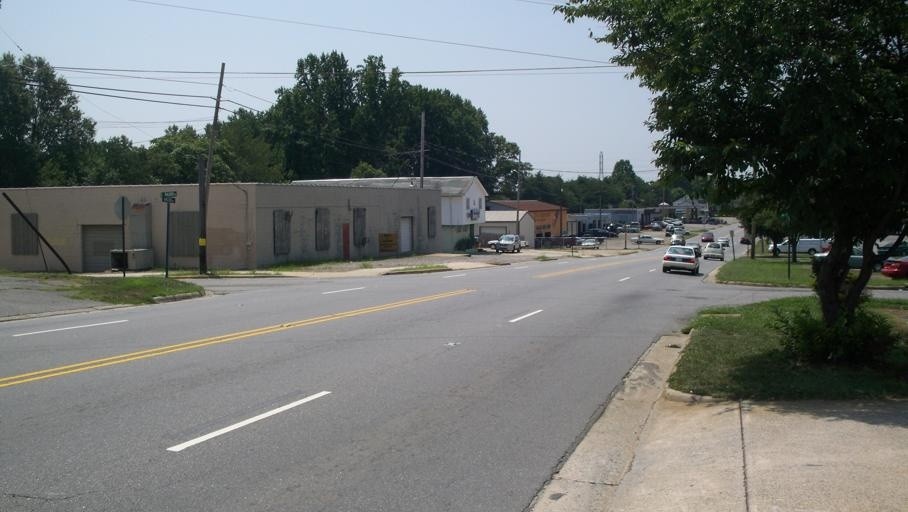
left=813, top=246, right=894, bottom=271
left=496, top=235, right=520, bottom=253
left=630, top=234, right=664, bottom=244
left=649, top=216, right=729, bottom=261
left=662, top=245, right=699, bottom=275
left=543, top=221, right=639, bottom=249
left=882, top=257, right=908, bottom=278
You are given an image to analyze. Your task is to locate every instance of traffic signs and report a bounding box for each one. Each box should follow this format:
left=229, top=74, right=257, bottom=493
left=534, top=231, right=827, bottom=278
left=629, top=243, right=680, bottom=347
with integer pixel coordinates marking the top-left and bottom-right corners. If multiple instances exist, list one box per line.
left=160, top=191, right=177, bottom=197
left=162, top=197, right=176, bottom=203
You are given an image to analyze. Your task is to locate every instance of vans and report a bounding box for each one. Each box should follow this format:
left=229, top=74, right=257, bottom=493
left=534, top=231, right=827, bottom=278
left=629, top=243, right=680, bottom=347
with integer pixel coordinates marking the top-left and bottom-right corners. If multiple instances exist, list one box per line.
left=769, top=238, right=826, bottom=255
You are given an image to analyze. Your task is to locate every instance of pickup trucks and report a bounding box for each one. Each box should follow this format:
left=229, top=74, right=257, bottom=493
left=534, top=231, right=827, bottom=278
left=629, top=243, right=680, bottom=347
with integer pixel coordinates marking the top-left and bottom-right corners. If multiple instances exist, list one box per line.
left=488, top=239, right=527, bottom=248
